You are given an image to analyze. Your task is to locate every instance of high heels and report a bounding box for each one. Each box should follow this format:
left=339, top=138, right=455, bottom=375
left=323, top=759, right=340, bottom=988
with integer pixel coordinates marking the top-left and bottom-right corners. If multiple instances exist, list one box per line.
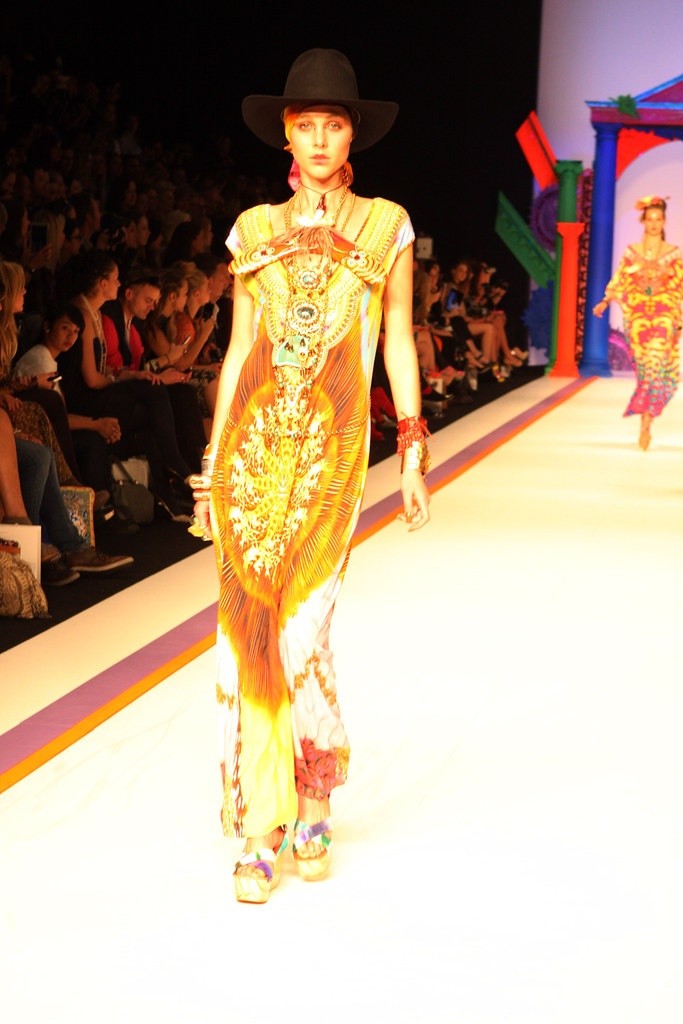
left=153, top=490, right=193, bottom=524
left=293, top=816, right=333, bottom=882
left=234, top=822, right=287, bottom=904
left=166, top=464, right=198, bottom=487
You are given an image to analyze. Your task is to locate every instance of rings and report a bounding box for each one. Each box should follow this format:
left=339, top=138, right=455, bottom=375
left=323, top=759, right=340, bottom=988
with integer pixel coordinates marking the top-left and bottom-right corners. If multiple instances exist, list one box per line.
left=198, top=524, right=208, bottom=530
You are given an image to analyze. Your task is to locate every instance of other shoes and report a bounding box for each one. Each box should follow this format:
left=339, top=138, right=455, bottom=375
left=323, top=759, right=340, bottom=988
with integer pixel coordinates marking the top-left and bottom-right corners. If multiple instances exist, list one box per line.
left=41, top=543, right=61, bottom=564
left=639, top=431, right=652, bottom=448
left=426, top=344, right=527, bottom=402
left=93, top=489, right=111, bottom=512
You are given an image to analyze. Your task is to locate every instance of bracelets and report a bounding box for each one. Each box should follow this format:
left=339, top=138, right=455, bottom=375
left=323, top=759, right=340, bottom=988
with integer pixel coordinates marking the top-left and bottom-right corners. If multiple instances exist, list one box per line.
left=188, top=474, right=213, bottom=489
left=192, top=491, right=212, bottom=503
left=396, top=417, right=430, bottom=475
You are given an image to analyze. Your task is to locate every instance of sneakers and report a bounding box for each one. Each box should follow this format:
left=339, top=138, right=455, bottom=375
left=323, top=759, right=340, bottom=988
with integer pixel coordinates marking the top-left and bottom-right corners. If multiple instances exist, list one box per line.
left=67, top=545, right=133, bottom=574
left=40, top=560, right=79, bottom=586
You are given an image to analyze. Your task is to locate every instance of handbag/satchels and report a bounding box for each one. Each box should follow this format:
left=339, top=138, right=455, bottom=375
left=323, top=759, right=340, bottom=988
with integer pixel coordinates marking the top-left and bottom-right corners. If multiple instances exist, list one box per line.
left=111, top=478, right=154, bottom=524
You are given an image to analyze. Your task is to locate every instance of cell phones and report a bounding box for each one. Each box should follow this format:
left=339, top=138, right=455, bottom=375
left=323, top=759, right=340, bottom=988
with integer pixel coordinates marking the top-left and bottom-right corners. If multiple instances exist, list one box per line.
left=49, top=374, right=62, bottom=382
left=29, top=224, right=49, bottom=254
left=182, top=336, right=191, bottom=344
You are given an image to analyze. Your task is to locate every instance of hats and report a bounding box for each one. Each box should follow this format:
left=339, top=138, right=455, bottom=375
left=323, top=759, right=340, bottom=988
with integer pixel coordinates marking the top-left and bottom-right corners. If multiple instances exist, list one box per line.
left=243, top=48, right=397, bottom=153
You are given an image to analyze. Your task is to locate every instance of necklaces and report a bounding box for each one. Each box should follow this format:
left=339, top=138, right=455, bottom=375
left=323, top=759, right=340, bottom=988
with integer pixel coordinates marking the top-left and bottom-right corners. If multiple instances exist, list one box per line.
left=300, top=180, right=344, bottom=222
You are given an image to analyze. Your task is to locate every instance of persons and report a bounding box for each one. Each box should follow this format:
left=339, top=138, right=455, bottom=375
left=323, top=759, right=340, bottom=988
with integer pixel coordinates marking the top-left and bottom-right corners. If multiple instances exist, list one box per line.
left=183, top=49, right=431, bottom=906
left=592, top=196, right=683, bottom=450
left=0, top=45, right=534, bottom=592
